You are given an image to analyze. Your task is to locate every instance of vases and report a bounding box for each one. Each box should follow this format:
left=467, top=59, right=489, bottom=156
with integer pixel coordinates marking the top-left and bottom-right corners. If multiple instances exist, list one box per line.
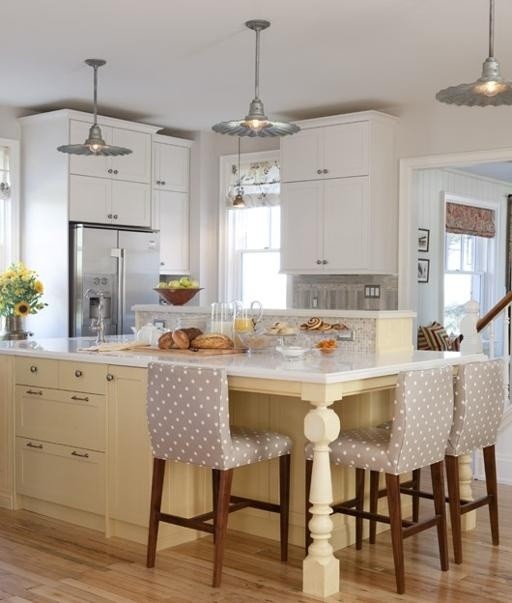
left=1, top=313, right=33, bottom=341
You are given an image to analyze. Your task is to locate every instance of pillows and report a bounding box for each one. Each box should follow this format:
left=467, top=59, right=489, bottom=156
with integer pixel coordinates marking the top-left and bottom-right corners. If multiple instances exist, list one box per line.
left=417, top=320, right=465, bottom=352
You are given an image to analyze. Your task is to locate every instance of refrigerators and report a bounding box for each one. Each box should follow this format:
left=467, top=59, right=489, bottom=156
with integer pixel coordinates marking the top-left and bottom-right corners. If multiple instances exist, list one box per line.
left=68, top=222, right=162, bottom=338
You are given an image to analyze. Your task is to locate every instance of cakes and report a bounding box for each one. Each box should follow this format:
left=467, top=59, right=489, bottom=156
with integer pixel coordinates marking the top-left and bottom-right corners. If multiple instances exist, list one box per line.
left=302, top=316, right=348, bottom=331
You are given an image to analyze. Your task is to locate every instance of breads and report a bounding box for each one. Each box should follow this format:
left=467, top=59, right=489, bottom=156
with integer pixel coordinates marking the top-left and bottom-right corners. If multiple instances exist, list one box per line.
left=159, top=328, right=234, bottom=350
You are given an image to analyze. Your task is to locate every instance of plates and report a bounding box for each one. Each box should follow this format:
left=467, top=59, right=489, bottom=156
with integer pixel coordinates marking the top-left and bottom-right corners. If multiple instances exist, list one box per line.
left=277, top=347, right=308, bottom=356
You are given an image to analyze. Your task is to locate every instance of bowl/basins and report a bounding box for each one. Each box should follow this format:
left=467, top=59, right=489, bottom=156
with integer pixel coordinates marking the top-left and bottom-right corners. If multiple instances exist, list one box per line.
left=313, top=347, right=341, bottom=358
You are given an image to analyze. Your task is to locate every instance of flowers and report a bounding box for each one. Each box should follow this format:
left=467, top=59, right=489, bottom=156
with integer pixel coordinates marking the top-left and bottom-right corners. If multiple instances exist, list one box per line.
left=1, top=260, right=50, bottom=319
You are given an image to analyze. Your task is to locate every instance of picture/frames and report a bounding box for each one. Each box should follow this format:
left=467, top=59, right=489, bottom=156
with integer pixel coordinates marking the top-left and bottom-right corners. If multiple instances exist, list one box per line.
left=417, top=228, right=431, bottom=282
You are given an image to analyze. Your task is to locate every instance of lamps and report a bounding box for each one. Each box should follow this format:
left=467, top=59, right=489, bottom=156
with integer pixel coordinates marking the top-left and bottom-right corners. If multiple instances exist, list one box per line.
left=54, top=60, right=133, bottom=158
left=434, top=0, right=512, bottom=109
left=210, top=21, right=301, bottom=138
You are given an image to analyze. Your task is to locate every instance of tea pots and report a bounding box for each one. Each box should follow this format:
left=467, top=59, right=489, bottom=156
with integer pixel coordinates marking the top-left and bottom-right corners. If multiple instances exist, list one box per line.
left=209, top=302, right=230, bottom=335
left=232, top=299, right=263, bottom=337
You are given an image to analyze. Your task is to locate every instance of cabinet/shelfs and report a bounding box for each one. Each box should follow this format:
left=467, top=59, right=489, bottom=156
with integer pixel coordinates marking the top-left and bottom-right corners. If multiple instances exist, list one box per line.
left=278, top=110, right=403, bottom=275
left=107, top=365, right=211, bottom=551
left=1, top=353, right=108, bottom=532
left=153, top=133, right=194, bottom=276
left=16, top=114, right=162, bottom=229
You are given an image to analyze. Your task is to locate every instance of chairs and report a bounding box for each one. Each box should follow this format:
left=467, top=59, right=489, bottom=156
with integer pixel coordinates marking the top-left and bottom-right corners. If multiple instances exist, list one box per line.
left=144, top=357, right=295, bottom=590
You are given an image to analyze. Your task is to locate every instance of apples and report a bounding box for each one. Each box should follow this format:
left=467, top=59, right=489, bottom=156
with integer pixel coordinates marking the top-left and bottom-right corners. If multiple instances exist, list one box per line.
left=158, top=276, right=200, bottom=289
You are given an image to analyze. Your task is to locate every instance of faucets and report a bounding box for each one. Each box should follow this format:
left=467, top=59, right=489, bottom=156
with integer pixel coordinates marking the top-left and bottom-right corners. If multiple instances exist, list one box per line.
left=82, top=285, right=106, bottom=344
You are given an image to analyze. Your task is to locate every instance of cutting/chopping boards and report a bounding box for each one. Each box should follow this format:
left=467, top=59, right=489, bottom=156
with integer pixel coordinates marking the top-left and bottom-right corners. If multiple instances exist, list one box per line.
left=133, top=343, right=246, bottom=356
